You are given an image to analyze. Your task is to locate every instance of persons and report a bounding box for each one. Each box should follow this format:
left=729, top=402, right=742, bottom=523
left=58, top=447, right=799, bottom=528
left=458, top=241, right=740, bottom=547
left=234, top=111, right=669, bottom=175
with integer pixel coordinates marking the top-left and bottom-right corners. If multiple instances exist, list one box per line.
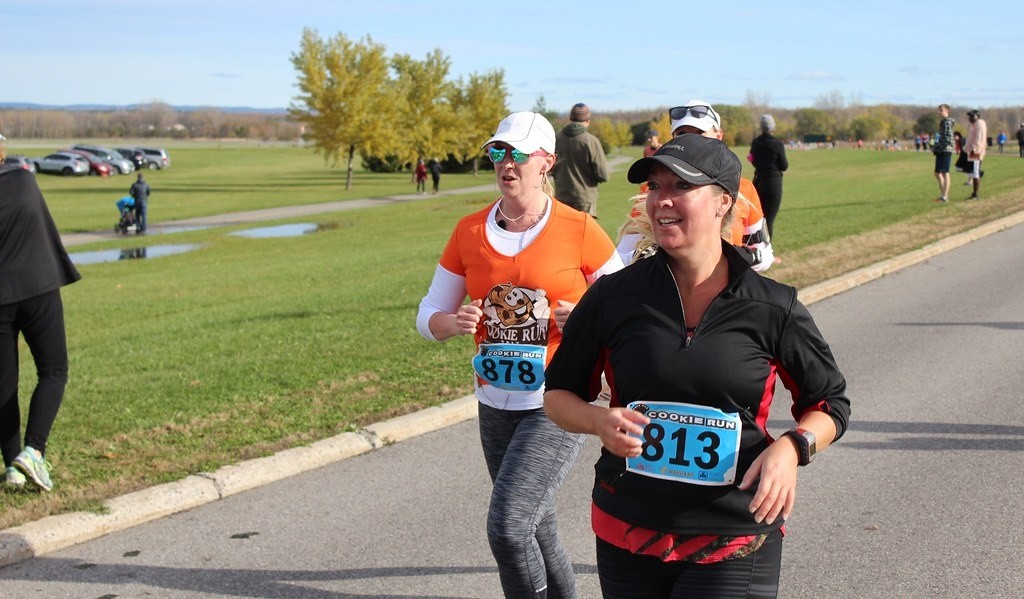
left=1, top=134, right=83, bottom=494
left=544, top=132, right=850, bottom=599
left=781, top=104, right=1024, bottom=204
left=114, top=172, right=150, bottom=233
left=431, top=157, right=442, bottom=194
left=554, top=103, right=609, bottom=221
left=415, top=112, right=626, bottom=599
left=747, top=114, right=788, bottom=265
left=633, top=97, right=774, bottom=273
left=414, top=159, right=429, bottom=194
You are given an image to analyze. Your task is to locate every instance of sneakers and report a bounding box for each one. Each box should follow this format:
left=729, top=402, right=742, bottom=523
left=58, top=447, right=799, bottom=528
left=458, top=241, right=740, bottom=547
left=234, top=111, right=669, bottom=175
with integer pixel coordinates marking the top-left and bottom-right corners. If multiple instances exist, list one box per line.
left=10, top=446, right=54, bottom=492
left=5, top=465, right=27, bottom=487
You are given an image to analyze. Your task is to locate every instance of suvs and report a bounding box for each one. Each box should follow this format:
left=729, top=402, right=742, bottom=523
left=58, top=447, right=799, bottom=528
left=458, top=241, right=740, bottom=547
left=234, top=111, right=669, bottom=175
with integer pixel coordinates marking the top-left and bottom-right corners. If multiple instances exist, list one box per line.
left=0, top=153, right=35, bottom=174
left=33, top=152, right=90, bottom=176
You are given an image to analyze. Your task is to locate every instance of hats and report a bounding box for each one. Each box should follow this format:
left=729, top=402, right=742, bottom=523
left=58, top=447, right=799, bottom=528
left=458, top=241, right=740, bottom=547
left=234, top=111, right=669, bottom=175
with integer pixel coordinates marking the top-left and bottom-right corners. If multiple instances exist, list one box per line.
left=670, top=99, right=722, bottom=135
left=626, top=133, right=743, bottom=214
left=758, top=114, right=776, bottom=132
left=642, top=129, right=659, bottom=138
left=568, top=103, right=592, bottom=121
left=966, top=110, right=982, bottom=118
left=479, top=110, right=557, bottom=156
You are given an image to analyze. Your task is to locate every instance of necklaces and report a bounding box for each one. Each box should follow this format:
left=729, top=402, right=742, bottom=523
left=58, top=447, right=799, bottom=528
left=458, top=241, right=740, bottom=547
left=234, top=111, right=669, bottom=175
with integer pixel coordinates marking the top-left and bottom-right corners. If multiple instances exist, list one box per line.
left=494, top=194, right=533, bottom=221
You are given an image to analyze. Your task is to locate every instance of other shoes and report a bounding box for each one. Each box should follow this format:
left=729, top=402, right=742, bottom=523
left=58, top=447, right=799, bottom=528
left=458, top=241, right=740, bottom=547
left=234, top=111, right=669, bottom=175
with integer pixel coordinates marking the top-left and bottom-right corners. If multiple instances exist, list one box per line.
left=964, top=194, right=979, bottom=203
left=934, top=196, right=950, bottom=204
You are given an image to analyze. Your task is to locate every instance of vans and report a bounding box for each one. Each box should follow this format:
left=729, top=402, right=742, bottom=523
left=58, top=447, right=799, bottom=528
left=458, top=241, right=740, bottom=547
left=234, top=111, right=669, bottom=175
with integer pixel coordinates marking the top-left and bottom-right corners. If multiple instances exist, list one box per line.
left=135, top=146, right=168, bottom=170
left=75, top=145, right=135, bottom=175
left=62, top=150, right=113, bottom=176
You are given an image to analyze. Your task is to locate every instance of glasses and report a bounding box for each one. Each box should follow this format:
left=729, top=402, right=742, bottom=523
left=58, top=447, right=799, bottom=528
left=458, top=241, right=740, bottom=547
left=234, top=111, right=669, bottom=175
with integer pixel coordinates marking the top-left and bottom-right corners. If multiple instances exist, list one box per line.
left=485, top=144, right=549, bottom=168
left=668, top=105, right=720, bottom=126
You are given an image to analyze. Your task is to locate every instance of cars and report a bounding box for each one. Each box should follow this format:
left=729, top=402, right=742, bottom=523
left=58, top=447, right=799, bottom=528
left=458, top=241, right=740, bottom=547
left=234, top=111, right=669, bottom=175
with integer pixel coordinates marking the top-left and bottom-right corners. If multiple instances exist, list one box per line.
left=116, top=148, right=143, bottom=171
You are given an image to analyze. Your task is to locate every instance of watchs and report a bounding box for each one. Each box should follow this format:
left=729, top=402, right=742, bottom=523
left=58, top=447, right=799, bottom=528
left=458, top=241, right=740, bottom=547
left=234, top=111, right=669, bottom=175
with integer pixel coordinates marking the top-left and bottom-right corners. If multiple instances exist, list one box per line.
left=779, top=427, right=817, bottom=466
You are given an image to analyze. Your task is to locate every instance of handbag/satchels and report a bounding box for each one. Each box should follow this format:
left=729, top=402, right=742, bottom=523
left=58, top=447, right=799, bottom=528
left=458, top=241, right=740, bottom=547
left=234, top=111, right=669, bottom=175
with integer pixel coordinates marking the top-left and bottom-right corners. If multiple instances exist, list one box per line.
left=961, top=158, right=981, bottom=181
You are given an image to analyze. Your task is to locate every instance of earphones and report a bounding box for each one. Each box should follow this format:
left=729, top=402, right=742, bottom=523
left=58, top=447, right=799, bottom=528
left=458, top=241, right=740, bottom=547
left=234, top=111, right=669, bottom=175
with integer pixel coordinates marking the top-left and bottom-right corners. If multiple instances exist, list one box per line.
left=542, top=162, right=549, bottom=171
